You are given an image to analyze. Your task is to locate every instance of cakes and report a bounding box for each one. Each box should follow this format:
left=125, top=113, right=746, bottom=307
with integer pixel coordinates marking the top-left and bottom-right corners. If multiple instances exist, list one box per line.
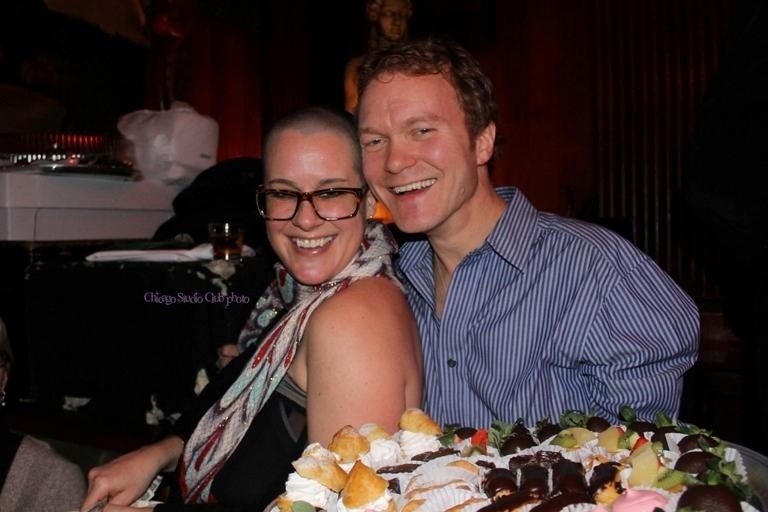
left=266, top=407, right=762, bottom=512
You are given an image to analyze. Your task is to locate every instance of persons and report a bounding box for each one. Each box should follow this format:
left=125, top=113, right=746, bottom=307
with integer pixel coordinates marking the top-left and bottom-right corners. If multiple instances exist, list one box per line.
left=354, top=28, right=702, bottom=422
left=1, top=104, right=425, bottom=511
left=342, top=0, right=415, bottom=116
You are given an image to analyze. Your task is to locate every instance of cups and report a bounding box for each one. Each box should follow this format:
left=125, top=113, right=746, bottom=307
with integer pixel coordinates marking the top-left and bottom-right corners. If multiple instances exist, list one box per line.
left=208, top=220, right=243, bottom=265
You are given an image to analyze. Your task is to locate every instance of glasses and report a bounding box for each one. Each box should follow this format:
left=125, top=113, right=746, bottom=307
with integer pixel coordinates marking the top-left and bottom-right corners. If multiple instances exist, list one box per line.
left=255, top=183, right=369, bottom=221
